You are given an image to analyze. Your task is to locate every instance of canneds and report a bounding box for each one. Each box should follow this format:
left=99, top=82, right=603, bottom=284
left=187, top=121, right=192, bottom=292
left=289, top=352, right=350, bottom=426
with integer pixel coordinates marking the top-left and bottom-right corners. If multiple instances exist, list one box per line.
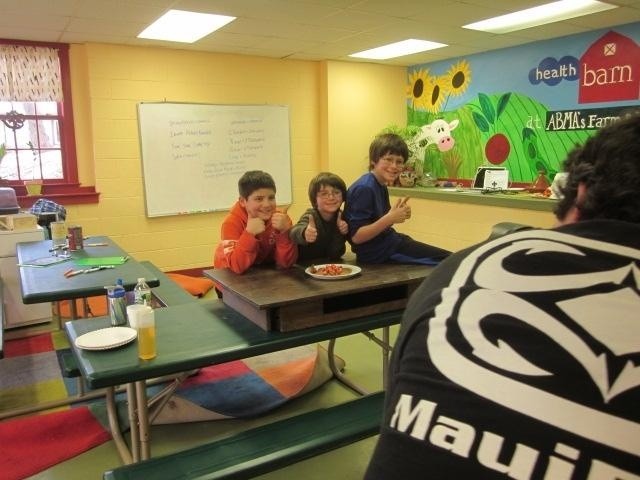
left=68, top=227, right=83, bottom=250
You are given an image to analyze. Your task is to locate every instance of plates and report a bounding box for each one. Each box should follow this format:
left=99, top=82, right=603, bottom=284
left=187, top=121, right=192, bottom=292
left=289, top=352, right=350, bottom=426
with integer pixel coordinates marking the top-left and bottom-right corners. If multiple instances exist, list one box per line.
left=304, top=263, right=364, bottom=281
left=73, top=326, right=137, bottom=352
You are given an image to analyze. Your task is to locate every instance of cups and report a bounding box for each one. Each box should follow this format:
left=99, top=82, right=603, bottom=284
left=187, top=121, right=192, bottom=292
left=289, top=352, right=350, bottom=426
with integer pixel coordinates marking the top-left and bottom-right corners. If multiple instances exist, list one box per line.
left=127, top=305, right=145, bottom=328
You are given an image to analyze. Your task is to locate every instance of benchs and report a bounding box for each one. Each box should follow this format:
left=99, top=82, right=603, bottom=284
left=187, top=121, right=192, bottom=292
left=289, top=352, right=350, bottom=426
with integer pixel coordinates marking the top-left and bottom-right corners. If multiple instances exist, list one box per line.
left=136, top=262, right=199, bottom=308
left=83, top=392, right=383, bottom=479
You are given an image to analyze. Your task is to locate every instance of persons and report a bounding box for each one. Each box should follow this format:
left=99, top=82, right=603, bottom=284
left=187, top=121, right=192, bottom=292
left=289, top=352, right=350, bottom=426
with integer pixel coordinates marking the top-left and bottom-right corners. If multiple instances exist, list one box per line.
left=364, top=115, right=640, bottom=480
left=212, top=170, right=298, bottom=299
left=343, top=134, right=454, bottom=267
left=289, top=172, right=349, bottom=266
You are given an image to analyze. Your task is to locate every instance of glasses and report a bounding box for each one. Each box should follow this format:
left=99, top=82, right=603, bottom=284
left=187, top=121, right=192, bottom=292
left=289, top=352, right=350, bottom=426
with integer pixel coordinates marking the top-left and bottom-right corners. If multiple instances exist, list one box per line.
left=317, top=191, right=342, bottom=199
left=383, top=156, right=403, bottom=165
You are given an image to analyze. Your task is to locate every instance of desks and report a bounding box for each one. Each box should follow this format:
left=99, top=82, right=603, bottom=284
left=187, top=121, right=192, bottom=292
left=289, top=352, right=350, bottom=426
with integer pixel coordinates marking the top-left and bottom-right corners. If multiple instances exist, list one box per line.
left=66, top=255, right=440, bottom=464
left=17, top=236, right=162, bottom=330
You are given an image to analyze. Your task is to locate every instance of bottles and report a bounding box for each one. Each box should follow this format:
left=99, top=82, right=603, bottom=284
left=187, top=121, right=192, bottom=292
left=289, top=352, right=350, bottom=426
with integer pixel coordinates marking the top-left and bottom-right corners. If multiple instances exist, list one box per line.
left=101, top=277, right=128, bottom=326
left=134, top=277, right=153, bottom=304
left=137, top=299, right=157, bottom=361
